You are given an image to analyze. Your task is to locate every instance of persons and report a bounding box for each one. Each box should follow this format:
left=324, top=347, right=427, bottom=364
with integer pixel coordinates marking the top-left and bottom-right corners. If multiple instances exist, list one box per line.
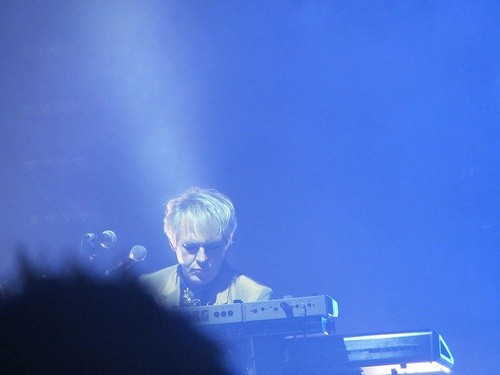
left=137, top=186, right=274, bottom=307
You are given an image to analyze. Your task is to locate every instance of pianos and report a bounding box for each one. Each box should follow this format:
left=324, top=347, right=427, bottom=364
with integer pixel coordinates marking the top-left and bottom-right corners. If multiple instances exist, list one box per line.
left=340, top=328, right=455, bottom=374
left=171, top=294, right=339, bottom=340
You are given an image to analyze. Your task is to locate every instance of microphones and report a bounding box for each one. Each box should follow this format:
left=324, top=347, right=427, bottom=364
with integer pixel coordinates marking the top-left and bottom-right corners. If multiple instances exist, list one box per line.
left=104, top=244, right=147, bottom=281
left=83, top=229, right=117, bottom=248
